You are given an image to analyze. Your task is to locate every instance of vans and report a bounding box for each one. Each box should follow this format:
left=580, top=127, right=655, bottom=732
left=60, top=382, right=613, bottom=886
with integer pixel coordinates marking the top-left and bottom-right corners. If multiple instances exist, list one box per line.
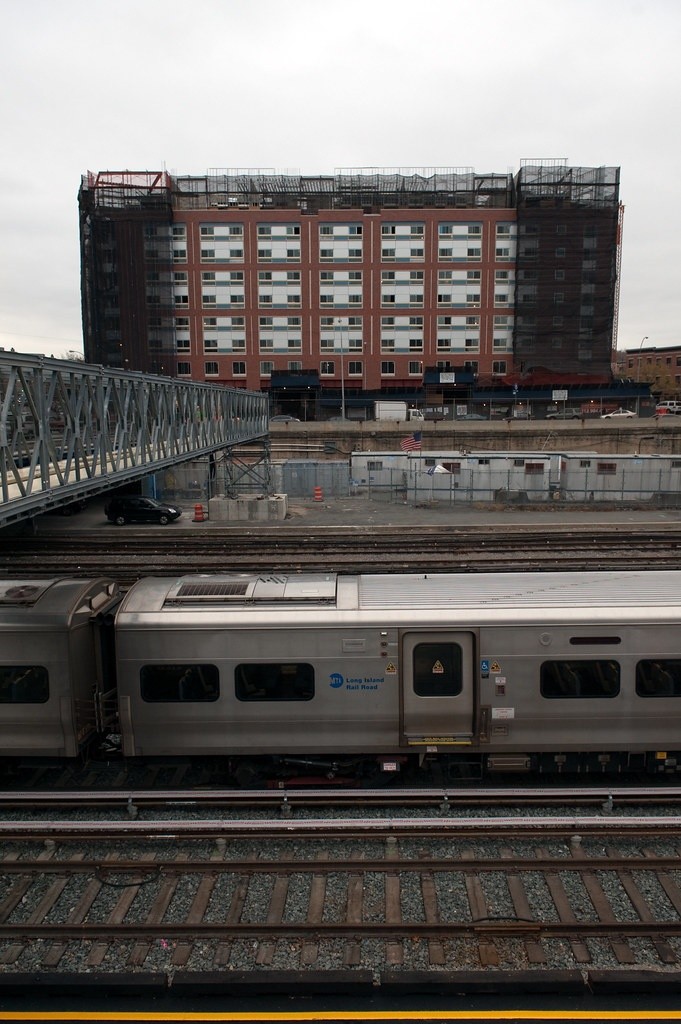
left=424, top=412, right=447, bottom=420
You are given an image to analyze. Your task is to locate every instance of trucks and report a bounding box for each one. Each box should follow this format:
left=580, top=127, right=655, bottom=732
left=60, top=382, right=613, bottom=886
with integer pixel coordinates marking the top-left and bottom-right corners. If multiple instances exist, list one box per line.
left=374, top=401, right=424, bottom=422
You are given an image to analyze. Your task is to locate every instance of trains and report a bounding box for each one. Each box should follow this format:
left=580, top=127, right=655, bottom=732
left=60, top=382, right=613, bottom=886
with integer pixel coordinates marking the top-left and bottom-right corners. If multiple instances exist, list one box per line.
left=0, top=571, right=681, bottom=791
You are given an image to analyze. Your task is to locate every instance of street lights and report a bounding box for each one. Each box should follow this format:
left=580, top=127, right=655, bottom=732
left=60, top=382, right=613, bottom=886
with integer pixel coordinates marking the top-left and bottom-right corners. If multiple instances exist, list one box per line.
left=338, top=316, right=345, bottom=417
left=638, top=337, right=648, bottom=381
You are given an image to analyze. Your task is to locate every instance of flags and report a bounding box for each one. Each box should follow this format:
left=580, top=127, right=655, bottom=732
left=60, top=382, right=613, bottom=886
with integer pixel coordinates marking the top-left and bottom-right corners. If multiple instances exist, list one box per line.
left=400, top=432, right=422, bottom=453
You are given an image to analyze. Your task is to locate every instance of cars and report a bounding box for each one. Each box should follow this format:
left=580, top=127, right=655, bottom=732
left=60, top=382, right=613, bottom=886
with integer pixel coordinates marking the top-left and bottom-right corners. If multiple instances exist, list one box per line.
left=655, top=400, right=681, bottom=413
left=600, top=407, right=637, bottom=419
left=45, top=494, right=87, bottom=515
left=104, top=496, right=181, bottom=526
left=502, top=412, right=531, bottom=420
left=545, top=408, right=583, bottom=420
left=456, top=414, right=487, bottom=420
left=269, top=415, right=299, bottom=424
left=325, top=417, right=351, bottom=422
left=6, top=406, right=64, bottom=440
